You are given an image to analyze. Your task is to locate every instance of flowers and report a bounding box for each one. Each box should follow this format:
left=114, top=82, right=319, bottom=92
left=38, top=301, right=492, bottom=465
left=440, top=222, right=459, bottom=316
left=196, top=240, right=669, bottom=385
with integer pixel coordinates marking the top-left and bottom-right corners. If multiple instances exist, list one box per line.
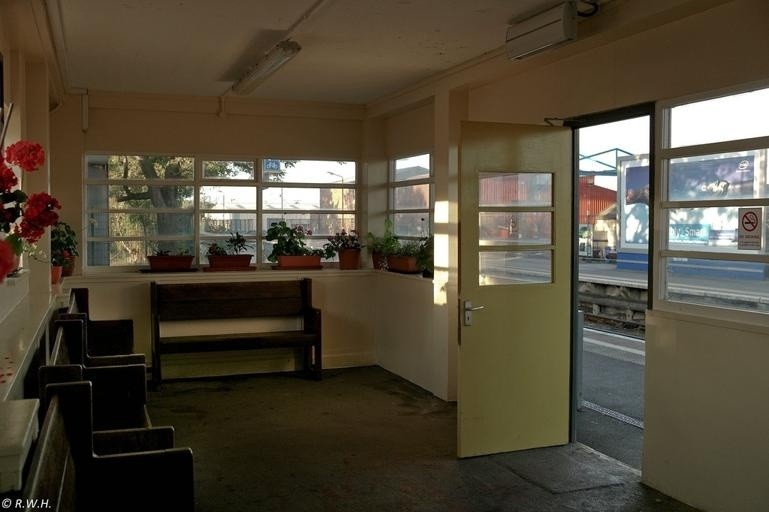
left=0, top=138, right=62, bottom=282
left=204, top=232, right=250, bottom=255
left=326, top=230, right=366, bottom=258
left=368, top=220, right=392, bottom=270
left=262, top=222, right=324, bottom=262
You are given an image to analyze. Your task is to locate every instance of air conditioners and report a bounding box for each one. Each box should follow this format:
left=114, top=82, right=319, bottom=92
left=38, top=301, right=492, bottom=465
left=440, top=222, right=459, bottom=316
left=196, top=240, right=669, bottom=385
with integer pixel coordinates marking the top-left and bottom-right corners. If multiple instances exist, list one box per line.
left=505, top=1, right=577, bottom=63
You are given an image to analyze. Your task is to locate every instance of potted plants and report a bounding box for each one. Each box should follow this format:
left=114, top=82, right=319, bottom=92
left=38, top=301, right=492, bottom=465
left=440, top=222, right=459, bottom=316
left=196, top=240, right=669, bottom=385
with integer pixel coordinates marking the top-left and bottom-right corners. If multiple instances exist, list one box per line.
left=51, top=229, right=63, bottom=284
left=61, top=221, right=80, bottom=277
left=386, top=232, right=420, bottom=274
left=416, top=235, right=434, bottom=278
left=147, top=240, right=195, bottom=272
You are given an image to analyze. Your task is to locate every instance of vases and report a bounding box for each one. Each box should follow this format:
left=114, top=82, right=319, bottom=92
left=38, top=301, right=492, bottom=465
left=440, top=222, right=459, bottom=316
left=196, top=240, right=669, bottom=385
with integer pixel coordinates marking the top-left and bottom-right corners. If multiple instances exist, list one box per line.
left=0, top=239, right=21, bottom=281
left=278, top=255, right=322, bottom=267
left=208, top=255, right=253, bottom=269
left=338, top=249, right=361, bottom=269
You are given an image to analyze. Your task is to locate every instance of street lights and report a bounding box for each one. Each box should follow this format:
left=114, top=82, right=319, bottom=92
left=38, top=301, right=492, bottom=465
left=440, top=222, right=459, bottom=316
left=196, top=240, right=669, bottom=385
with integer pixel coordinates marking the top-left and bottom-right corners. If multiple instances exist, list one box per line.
left=218, top=191, right=225, bottom=229
left=327, top=171, right=345, bottom=229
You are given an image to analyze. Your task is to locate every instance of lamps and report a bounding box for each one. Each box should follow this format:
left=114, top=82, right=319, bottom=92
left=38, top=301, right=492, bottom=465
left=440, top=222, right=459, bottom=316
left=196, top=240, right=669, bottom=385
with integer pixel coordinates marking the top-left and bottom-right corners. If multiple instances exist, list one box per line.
left=231, top=38, right=301, bottom=95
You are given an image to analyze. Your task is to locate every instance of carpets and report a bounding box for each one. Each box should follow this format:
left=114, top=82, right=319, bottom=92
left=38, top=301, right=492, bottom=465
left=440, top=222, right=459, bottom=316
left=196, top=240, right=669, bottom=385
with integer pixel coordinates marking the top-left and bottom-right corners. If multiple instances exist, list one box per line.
left=492, top=442, right=643, bottom=495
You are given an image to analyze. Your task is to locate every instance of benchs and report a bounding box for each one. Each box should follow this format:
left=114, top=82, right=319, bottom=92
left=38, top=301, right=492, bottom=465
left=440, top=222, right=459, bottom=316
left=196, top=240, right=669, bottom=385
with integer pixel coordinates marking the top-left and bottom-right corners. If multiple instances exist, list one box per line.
left=148, top=277, right=321, bottom=392
left=55, top=288, right=146, bottom=364
left=20, top=381, right=194, bottom=512
left=40, top=320, right=176, bottom=456
